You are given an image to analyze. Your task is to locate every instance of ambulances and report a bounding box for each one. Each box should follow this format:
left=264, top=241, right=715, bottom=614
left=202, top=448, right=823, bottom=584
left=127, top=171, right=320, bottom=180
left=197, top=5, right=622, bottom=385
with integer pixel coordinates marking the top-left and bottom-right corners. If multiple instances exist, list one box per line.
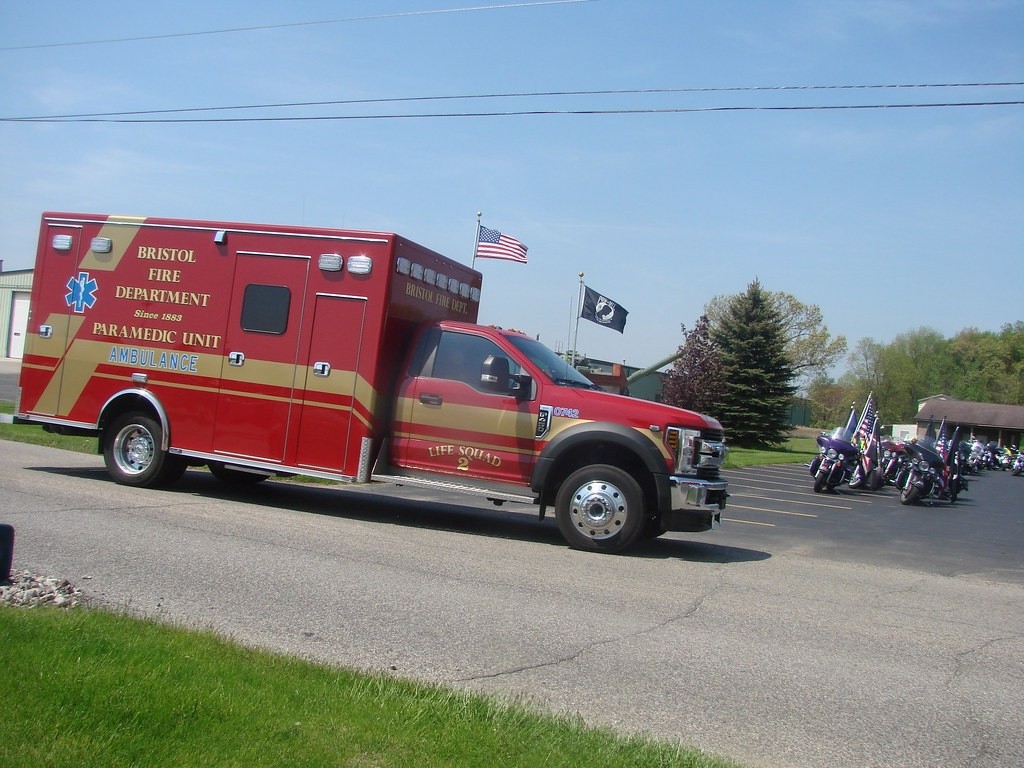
left=12, top=211, right=732, bottom=555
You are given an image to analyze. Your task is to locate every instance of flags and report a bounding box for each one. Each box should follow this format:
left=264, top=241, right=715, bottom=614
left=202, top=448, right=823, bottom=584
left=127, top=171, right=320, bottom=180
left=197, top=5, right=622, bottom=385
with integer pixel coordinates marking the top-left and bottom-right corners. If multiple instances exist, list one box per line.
left=476, top=225, right=528, bottom=264
left=843, top=395, right=880, bottom=476
left=580, top=285, right=628, bottom=334
left=925, top=418, right=961, bottom=496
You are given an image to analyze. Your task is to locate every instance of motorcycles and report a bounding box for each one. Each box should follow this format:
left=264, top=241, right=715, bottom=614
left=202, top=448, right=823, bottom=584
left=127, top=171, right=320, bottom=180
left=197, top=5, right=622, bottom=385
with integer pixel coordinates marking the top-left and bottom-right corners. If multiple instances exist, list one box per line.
left=804, top=426, right=1024, bottom=506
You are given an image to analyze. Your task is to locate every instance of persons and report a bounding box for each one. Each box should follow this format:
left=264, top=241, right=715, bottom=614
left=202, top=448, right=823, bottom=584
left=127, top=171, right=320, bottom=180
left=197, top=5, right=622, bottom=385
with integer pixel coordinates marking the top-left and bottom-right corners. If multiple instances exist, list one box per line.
left=971, top=440, right=984, bottom=452
